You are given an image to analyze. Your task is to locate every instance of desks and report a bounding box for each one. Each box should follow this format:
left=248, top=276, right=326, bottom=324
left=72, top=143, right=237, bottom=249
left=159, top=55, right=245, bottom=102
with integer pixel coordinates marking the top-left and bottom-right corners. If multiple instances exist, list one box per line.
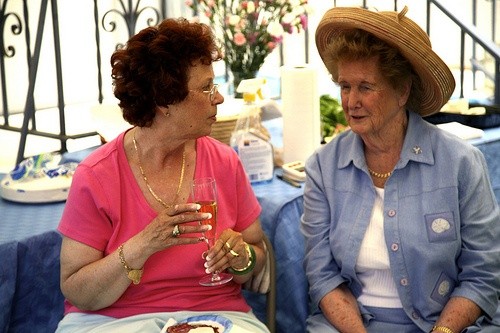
left=243, top=117, right=500, bottom=333
left=0, top=144, right=101, bottom=333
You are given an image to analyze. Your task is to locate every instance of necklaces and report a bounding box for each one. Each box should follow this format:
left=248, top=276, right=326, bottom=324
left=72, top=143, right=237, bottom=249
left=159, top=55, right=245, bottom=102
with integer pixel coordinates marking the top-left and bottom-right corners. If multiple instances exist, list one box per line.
left=127, top=125, right=186, bottom=210
left=364, top=162, right=395, bottom=179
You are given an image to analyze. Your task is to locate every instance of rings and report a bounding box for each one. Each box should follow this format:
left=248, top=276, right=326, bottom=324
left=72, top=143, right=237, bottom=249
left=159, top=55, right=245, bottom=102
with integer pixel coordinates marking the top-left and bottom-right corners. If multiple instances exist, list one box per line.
left=172, top=223, right=180, bottom=236
left=225, top=241, right=232, bottom=249
left=229, top=250, right=239, bottom=257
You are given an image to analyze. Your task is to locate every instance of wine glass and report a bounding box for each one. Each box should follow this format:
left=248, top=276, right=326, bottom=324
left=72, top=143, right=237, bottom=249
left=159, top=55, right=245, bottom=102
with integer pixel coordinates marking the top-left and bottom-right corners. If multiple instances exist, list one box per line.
left=192, top=177, right=234, bottom=286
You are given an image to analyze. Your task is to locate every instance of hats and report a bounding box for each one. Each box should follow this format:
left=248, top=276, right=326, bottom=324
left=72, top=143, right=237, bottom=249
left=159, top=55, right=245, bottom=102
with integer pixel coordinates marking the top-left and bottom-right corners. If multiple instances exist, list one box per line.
left=315, top=6, right=456, bottom=119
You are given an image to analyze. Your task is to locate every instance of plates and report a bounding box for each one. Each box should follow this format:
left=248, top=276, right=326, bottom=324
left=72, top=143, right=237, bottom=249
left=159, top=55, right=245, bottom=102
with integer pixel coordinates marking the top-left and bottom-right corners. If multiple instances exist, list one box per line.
left=177, top=314, right=232, bottom=333
left=0, top=174, right=72, bottom=204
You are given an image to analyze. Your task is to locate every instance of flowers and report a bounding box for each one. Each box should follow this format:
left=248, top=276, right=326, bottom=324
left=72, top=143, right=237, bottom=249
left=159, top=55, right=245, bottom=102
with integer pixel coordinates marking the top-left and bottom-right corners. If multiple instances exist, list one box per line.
left=184, top=0, right=309, bottom=98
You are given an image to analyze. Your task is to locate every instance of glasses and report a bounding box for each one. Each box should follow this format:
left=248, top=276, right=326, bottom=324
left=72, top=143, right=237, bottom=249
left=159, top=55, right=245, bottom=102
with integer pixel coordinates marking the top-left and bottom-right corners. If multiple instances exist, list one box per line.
left=188, top=84, right=219, bottom=102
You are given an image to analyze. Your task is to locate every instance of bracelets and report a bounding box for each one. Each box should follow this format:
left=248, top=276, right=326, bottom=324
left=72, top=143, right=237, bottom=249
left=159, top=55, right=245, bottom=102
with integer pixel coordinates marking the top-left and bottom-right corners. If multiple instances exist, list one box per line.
left=432, top=325, right=455, bottom=333
left=229, top=242, right=256, bottom=275
left=117, top=242, right=145, bottom=285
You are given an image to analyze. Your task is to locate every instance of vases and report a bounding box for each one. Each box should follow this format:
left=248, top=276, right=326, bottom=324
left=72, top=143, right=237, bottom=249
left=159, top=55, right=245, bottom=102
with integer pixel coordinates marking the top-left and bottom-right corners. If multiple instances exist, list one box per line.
left=218, top=68, right=272, bottom=99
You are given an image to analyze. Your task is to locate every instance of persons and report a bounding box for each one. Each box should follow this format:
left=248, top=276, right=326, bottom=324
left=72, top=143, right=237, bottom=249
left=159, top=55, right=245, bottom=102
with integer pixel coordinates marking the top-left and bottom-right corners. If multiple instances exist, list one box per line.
left=296, top=7, right=500, bottom=333
left=54, top=16, right=271, bottom=333
